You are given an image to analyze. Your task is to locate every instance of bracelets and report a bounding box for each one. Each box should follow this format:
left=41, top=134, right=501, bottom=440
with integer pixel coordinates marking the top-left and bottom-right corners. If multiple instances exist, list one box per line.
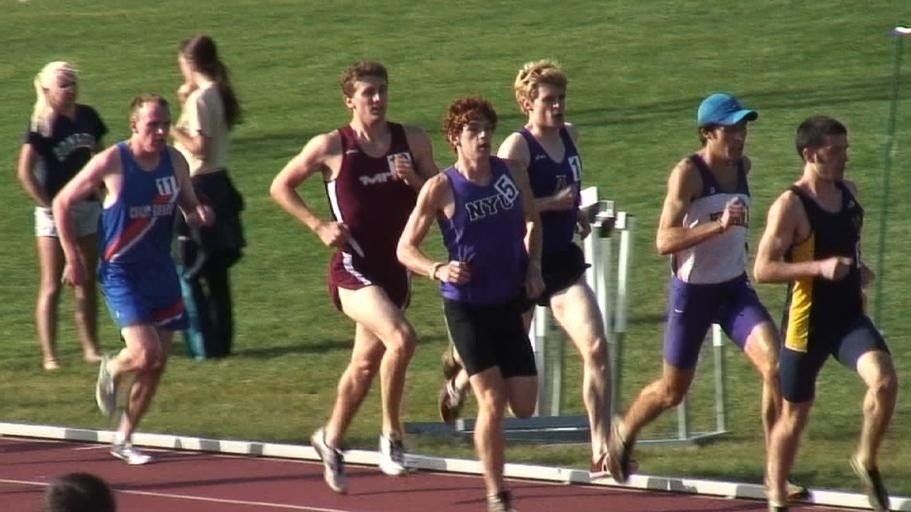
left=529, top=258, right=544, bottom=270
left=717, top=218, right=725, bottom=233
left=430, top=261, right=444, bottom=279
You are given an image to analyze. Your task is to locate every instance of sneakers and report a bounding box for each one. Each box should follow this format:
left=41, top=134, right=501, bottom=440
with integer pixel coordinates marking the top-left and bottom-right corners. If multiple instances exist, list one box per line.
left=379, top=426, right=417, bottom=475
left=108, top=442, right=153, bottom=465
left=96, top=349, right=122, bottom=420
left=310, top=425, right=348, bottom=493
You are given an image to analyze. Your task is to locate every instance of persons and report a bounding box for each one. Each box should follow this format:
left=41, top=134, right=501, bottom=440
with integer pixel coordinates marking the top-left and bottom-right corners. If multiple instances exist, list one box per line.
left=397, top=96, right=549, bottom=510
left=169, top=34, right=244, bottom=362
left=607, top=95, right=809, bottom=506
left=16, top=60, right=108, bottom=370
left=269, top=60, right=442, bottom=496
left=757, top=113, right=899, bottom=512
left=45, top=473, right=117, bottom=512
left=440, top=60, right=638, bottom=481
left=52, top=92, right=216, bottom=465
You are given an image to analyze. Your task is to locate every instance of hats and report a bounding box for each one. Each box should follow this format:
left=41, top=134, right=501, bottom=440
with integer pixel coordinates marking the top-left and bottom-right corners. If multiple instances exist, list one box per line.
left=696, top=95, right=760, bottom=129
